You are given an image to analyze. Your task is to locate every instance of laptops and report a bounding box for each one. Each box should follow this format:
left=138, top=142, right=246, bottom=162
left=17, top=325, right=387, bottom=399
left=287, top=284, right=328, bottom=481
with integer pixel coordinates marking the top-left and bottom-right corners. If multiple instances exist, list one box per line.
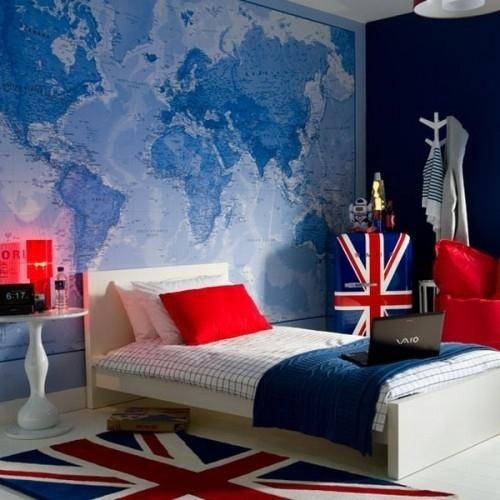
left=341, top=311, right=446, bottom=367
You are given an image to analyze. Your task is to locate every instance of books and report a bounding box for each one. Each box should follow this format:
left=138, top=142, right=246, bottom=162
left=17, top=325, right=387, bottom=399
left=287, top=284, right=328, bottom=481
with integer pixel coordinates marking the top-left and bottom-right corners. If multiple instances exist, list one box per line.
left=107, top=404, right=192, bottom=434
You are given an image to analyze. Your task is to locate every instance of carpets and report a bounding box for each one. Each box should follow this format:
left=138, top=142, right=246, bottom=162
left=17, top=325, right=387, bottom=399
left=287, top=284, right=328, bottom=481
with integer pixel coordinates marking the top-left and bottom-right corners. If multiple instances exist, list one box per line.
left=0, top=428, right=455, bottom=500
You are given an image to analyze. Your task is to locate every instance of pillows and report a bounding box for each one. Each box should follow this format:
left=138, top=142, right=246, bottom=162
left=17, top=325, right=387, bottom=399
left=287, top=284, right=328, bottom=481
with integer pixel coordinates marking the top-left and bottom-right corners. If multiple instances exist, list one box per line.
left=114, top=279, right=272, bottom=347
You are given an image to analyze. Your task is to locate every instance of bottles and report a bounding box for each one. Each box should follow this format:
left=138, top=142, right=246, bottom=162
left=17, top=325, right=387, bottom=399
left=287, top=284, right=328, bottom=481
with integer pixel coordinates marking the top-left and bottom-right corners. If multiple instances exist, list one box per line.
left=386, top=200, right=397, bottom=231
left=370, top=172, right=387, bottom=232
left=53, top=265, right=69, bottom=314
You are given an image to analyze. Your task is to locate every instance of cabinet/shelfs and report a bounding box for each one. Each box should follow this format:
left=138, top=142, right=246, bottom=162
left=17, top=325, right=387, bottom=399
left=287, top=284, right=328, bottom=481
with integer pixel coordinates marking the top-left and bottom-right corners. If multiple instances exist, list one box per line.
left=325, top=234, right=415, bottom=337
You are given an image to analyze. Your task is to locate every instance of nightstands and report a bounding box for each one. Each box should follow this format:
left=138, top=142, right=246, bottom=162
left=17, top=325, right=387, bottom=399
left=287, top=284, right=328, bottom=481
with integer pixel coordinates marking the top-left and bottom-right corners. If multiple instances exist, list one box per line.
left=0, top=308, right=88, bottom=441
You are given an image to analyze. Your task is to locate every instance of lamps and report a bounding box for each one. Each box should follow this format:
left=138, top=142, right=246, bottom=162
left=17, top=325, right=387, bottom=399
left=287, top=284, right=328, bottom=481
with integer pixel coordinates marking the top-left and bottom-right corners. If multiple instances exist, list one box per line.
left=26, top=238, right=52, bottom=309
left=413, top=0, right=500, bottom=20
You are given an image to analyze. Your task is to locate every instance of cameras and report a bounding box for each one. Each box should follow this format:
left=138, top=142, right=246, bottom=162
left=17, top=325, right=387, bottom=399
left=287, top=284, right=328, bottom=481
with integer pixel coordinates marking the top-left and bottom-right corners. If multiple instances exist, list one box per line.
left=32, top=294, right=45, bottom=312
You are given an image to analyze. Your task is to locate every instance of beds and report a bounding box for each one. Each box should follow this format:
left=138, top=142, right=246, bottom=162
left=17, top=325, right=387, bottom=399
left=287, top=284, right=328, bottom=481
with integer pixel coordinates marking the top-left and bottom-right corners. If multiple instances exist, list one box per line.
left=86, top=266, right=500, bottom=486
